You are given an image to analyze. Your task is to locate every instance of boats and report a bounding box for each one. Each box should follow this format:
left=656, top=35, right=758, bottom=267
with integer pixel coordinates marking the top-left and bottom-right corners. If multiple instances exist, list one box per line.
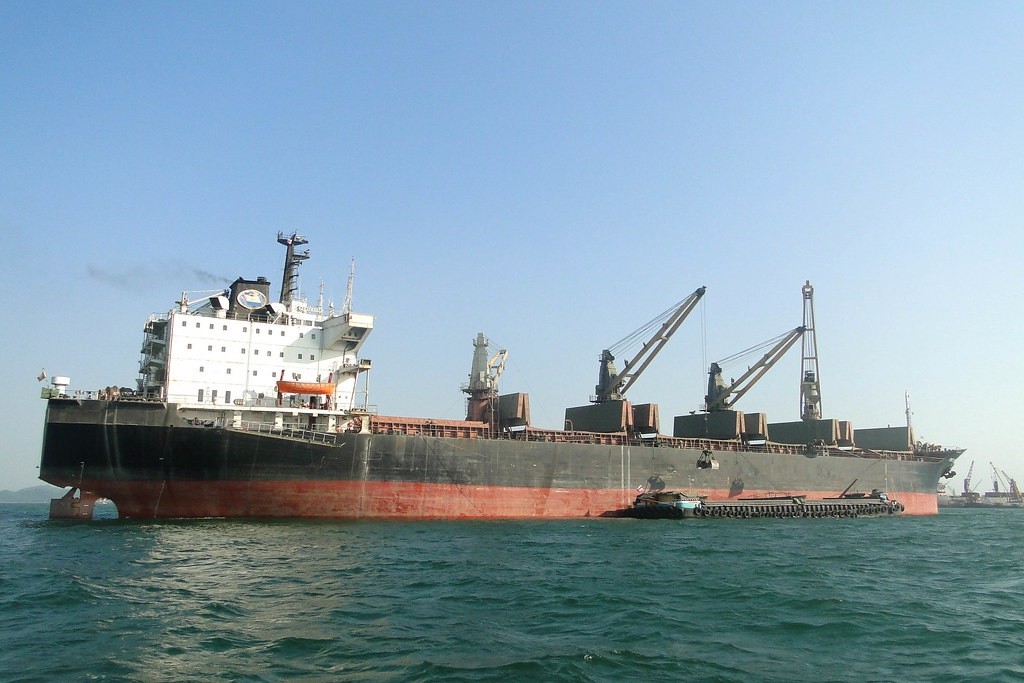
left=276, top=379, right=334, bottom=396
left=34, top=231, right=972, bottom=526
left=626, top=480, right=904, bottom=522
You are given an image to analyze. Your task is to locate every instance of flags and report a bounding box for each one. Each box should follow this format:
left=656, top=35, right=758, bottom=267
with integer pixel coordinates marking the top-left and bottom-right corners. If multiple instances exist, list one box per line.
left=37, top=371, right=46, bottom=381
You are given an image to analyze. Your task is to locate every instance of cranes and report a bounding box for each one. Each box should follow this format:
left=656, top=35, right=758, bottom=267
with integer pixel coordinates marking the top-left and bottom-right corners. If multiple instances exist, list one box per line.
left=960, top=459, right=1023, bottom=502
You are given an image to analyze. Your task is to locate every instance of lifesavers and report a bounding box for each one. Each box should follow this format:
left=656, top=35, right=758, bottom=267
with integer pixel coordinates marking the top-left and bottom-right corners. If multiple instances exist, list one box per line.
left=693, top=503, right=905, bottom=519
left=620, top=505, right=685, bottom=520
